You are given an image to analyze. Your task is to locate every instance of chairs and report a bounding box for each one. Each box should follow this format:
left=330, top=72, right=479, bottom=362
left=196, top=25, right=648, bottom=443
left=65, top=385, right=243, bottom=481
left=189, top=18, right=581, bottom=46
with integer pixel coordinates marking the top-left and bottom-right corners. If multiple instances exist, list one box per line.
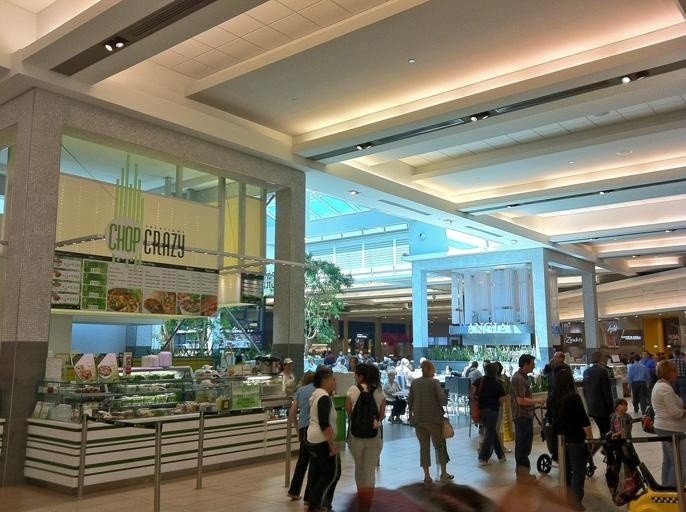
left=379, top=370, right=487, bottom=437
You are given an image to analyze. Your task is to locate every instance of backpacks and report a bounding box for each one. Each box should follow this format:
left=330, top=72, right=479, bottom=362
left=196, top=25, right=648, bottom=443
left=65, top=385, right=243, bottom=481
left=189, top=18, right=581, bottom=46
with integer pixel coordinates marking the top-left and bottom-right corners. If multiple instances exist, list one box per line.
left=350, top=384, right=379, bottom=438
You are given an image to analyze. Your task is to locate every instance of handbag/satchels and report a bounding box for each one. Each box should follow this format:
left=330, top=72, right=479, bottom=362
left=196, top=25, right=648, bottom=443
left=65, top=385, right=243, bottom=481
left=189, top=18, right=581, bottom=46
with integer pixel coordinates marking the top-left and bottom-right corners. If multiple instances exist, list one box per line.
left=641, top=405, right=654, bottom=434
left=442, top=418, right=454, bottom=439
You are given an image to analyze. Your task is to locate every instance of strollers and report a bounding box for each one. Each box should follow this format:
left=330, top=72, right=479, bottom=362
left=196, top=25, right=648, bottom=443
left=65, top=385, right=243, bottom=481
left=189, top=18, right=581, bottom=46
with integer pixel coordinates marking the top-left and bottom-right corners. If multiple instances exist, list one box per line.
left=530, top=403, right=595, bottom=478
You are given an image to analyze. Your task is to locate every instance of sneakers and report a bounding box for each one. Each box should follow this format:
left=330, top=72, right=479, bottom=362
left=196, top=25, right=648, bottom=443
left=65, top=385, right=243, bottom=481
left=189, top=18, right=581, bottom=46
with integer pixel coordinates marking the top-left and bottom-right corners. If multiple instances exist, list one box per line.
left=440, top=474, right=453, bottom=481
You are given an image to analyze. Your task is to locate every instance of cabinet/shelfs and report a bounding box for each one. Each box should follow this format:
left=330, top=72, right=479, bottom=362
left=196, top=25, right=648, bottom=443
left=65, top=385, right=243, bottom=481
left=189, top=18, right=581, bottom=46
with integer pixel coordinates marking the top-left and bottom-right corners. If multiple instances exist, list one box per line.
left=220, top=306, right=264, bottom=349
left=101, top=366, right=198, bottom=414
left=172, top=318, right=206, bottom=357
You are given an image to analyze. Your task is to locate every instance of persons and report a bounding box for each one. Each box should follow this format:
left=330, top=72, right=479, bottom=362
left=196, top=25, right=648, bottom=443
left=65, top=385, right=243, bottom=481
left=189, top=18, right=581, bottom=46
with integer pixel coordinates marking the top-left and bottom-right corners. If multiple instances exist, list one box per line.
left=344, top=363, right=386, bottom=511
left=303, top=349, right=426, bottom=424
left=200, top=371, right=213, bottom=385
left=407, top=360, right=454, bottom=487
left=444, top=349, right=685, bottom=512
left=279, top=358, right=295, bottom=382
left=304, top=369, right=341, bottom=511
left=286, top=372, right=317, bottom=498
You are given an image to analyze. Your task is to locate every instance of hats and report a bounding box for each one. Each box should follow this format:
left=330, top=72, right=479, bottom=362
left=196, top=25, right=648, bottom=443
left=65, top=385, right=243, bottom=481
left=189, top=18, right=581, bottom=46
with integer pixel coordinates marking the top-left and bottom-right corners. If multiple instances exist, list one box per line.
left=284, top=357, right=293, bottom=364
